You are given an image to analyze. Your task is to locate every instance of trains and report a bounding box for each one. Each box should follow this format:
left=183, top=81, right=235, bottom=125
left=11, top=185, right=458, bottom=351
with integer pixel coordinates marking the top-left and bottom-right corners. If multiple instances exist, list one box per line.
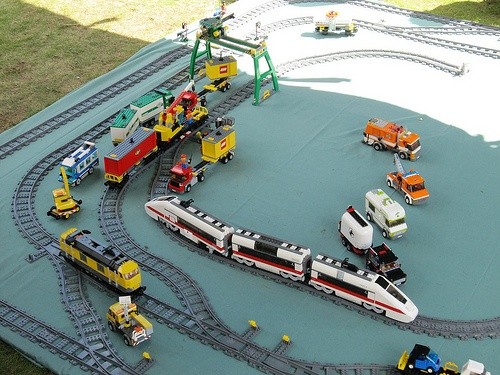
left=102, top=79, right=209, bottom=188
left=59, top=227, right=147, bottom=302
left=145, top=195, right=421, bottom=325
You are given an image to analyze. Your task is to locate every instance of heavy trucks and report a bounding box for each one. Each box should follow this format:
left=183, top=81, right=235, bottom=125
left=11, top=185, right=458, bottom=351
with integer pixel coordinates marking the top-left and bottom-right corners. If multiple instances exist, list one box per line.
left=167, top=125, right=237, bottom=195
left=180, top=55, right=238, bottom=111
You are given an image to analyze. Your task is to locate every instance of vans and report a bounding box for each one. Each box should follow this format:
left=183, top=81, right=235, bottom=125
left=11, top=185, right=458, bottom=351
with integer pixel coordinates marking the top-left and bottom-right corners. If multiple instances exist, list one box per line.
left=365, top=188, right=408, bottom=241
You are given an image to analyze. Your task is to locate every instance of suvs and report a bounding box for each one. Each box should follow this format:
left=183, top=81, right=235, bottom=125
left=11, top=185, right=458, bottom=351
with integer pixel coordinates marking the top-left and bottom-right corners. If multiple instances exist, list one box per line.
left=365, top=242, right=409, bottom=287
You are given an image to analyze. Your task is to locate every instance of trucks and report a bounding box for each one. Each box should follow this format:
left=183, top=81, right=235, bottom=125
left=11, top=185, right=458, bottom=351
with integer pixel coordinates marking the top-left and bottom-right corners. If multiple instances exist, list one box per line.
left=396, top=350, right=492, bottom=375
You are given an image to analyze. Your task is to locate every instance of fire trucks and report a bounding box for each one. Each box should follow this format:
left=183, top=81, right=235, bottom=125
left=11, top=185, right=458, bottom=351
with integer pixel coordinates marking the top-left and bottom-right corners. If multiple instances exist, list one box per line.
left=359, top=113, right=423, bottom=161
left=387, top=153, right=429, bottom=206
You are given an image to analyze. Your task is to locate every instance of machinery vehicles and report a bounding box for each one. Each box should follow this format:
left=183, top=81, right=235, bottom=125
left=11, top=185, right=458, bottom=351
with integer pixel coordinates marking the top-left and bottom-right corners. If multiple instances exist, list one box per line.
left=106, top=296, right=153, bottom=346
left=46, top=166, right=82, bottom=221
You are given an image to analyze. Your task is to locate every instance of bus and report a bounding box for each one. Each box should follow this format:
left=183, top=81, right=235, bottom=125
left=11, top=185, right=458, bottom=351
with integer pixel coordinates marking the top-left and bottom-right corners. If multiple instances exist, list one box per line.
left=57, top=140, right=100, bottom=186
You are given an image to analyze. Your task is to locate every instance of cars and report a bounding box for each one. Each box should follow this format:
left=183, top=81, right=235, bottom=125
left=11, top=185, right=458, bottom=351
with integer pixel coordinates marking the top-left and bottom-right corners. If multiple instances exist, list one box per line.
left=408, top=344, right=441, bottom=373
